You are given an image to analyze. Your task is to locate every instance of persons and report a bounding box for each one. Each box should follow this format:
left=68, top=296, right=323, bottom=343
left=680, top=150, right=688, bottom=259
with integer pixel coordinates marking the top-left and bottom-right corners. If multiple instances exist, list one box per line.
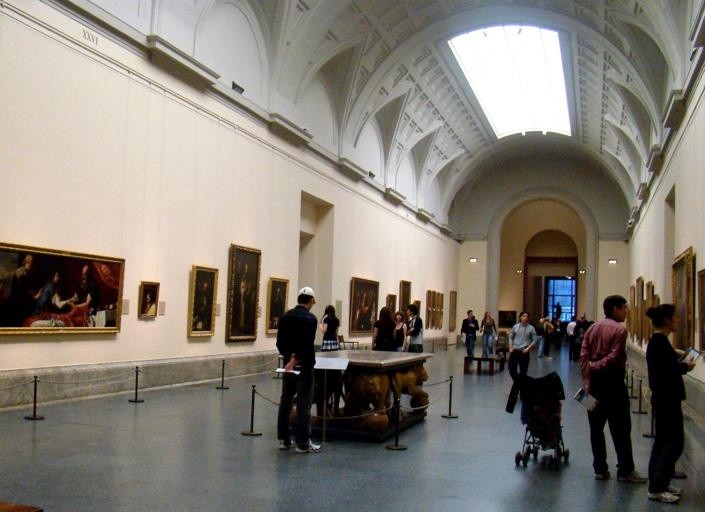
left=371, top=307, right=397, bottom=351
left=11, top=255, right=97, bottom=320
left=535, top=315, right=595, bottom=361
left=507, top=311, right=538, bottom=388
left=578, top=295, right=648, bottom=483
left=645, top=303, right=696, bottom=503
left=479, top=311, right=497, bottom=355
left=318, top=305, right=341, bottom=352
left=276, top=286, right=323, bottom=453
left=406, top=305, right=424, bottom=352
left=460, top=310, right=479, bottom=363
left=394, top=312, right=407, bottom=352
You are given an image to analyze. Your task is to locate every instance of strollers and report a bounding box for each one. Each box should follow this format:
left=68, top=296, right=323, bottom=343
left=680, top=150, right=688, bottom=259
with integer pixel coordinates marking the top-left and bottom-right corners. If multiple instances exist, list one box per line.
left=515, top=370, right=570, bottom=472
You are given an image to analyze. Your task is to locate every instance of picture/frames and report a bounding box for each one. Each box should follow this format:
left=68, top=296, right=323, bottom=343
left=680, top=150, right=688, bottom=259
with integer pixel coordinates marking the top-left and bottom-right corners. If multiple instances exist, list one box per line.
left=265, top=276, right=290, bottom=335
left=0, top=241, right=125, bottom=336
left=186, top=264, right=220, bottom=336
left=225, top=242, right=262, bottom=343
left=697, top=268, right=705, bottom=352
left=671, top=246, right=694, bottom=352
left=138, top=281, right=160, bottom=318
left=349, top=277, right=380, bottom=336
left=385, top=278, right=458, bottom=333
left=624, top=275, right=660, bottom=347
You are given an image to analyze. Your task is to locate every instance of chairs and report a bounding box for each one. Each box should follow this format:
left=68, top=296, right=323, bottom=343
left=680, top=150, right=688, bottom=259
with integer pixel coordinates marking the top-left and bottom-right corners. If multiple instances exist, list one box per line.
left=338, top=334, right=359, bottom=349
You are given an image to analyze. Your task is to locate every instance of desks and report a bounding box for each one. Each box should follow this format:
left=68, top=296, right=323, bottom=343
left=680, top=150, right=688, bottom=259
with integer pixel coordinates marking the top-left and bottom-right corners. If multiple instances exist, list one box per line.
left=312, top=349, right=435, bottom=442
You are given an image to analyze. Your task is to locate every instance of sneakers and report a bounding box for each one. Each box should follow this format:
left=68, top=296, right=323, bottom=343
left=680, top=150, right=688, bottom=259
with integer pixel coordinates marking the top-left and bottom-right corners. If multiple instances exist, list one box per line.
left=278, top=438, right=322, bottom=454
left=648, top=484, right=684, bottom=503
left=617, top=470, right=648, bottom=483
left=672, top=472, right=687, bottom=479
left=594, top=471, right=610, bottom=480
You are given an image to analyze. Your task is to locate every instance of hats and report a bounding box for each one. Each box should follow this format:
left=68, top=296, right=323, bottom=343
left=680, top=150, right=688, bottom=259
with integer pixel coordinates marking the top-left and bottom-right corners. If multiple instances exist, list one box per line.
left=297, top=287, right=320, bottom=304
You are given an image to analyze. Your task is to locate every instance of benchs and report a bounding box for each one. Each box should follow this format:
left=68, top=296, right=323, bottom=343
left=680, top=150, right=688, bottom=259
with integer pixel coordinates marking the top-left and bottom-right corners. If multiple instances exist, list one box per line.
left=464, top=355, right=505, bottom=376
